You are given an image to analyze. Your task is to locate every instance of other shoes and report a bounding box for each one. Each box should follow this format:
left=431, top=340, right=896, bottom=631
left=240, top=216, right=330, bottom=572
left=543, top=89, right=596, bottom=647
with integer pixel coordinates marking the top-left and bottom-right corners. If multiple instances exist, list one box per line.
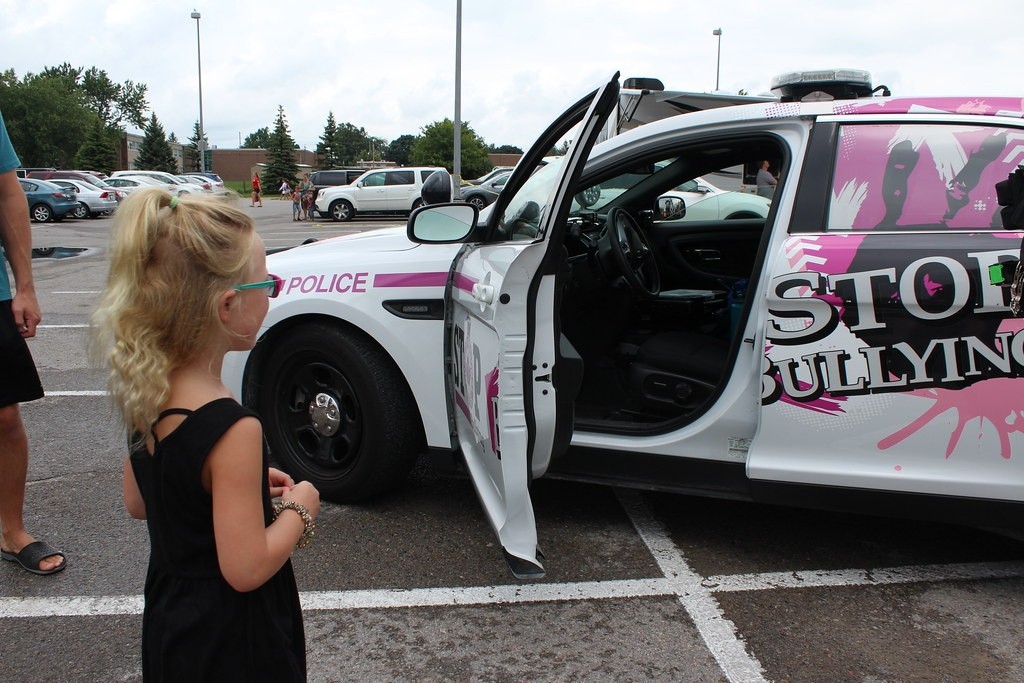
left=249, top=205, right=255, bottom=207
left=302, top=218, right=307, bottom=220
left=258, top=205, right=263, bottom=207
left=307, top=219, right=311, bottom=222
left=297, top=217, right=302, bottom=221
left=311, top=220, right=314, bottom=222
left=293, top=218, right=297, bottom=221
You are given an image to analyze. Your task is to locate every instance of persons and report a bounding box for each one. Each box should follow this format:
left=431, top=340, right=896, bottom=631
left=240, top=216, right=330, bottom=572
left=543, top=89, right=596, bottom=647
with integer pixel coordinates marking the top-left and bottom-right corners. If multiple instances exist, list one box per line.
left=248, top=172, right=263, bottom=207
left=291, top=173, right=317, bottom=222
left=84, top=184, right=321, bottom=683
left=279, top=180, right=293, bottom=200
left=756, top=160, right=778, bottom=200
left=0, top=113, right=67, bottom=577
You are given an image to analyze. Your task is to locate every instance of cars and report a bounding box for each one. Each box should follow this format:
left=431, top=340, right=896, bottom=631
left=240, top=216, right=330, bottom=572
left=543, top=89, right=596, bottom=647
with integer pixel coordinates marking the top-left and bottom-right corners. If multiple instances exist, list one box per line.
left=18, top=178, right=81, bottom=224
left=460, top=167, right=517, bottom=187
left=213, top=68, right=1024, bottom=580
left=15, top=167, right=109, bottom=183
left=460, top=171, right=517, bottom=214
left=41, top=178, right=118, bottom=220
left=101, top=176, right=177, bottom=203
left=467, top=155, right=774, bottom=227
left=177, top=172, right=225, bottom=196
left=24, top=171, right=124, bottom=209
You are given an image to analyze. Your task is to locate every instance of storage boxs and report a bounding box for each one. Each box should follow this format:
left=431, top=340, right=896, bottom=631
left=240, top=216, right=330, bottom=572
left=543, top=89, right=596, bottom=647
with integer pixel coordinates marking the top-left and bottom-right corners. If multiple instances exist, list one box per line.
left=644, top=290, right=729, bottom=330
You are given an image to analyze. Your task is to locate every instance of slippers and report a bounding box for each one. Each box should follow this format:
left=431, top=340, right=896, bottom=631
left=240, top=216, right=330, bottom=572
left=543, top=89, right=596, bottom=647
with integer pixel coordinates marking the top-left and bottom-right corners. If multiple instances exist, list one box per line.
left=0, top=541, right=67, bottom=574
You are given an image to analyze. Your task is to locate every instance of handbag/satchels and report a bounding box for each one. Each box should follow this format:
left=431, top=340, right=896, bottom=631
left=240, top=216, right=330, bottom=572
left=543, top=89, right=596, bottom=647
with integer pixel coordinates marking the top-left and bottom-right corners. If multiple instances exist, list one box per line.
left=251, top=192, right=259, bottom=202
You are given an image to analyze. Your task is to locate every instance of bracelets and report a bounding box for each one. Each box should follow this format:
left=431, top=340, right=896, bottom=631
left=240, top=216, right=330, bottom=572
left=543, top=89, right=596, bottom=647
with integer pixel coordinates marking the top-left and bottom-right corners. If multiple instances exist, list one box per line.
left=271, top=500, right=314, bottom=548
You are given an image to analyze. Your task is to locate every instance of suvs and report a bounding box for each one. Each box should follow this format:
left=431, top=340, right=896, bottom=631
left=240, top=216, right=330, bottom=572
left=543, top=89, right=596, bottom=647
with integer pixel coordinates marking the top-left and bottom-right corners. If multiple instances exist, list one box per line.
left=313, top=166, right=456, bottom=222
left=306, top=169, right=385, bottom=218
left=109, top=170, right=206, bottom=200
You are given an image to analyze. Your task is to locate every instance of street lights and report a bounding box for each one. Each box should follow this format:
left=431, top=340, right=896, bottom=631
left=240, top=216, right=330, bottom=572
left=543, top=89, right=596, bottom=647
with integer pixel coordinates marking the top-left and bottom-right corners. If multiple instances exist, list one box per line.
left=713, top=28, right=723, bottom=93
left=189, top=8, right=206, bottom=175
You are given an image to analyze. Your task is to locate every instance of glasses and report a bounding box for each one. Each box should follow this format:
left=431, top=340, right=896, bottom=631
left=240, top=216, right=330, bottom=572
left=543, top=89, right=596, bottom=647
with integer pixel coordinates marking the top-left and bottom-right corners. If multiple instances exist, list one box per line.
left=225, top=274, right=282, bottom=298
